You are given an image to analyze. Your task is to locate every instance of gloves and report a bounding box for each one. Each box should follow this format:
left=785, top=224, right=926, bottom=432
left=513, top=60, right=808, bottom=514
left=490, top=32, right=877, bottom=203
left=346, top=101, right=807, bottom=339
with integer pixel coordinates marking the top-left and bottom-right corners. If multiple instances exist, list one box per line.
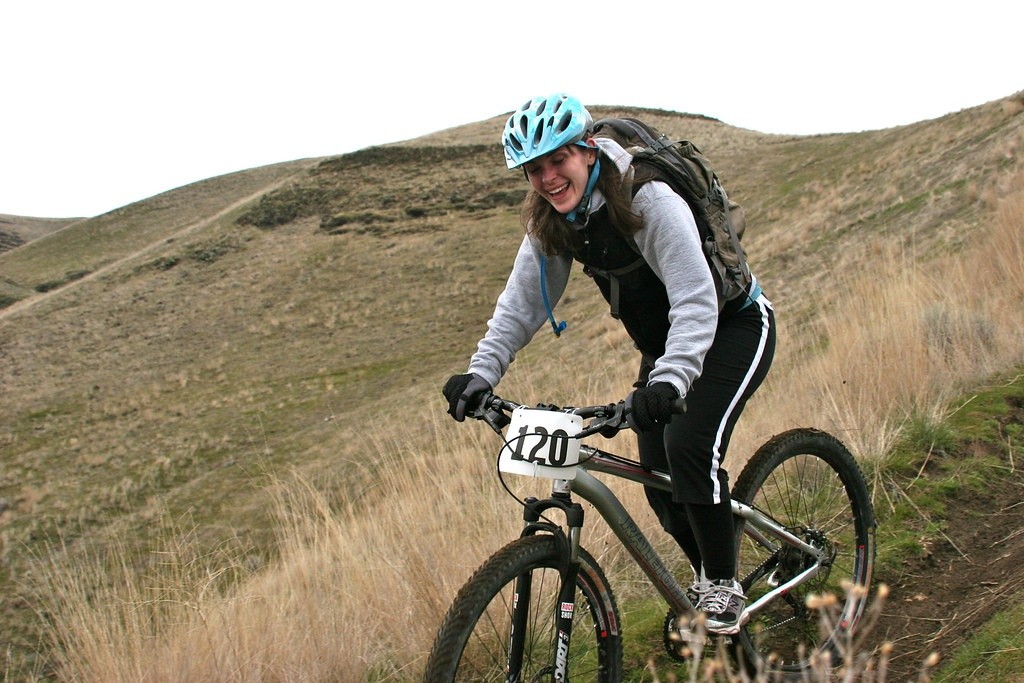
left=443, top=373, right=492, bottom=422
left=624, top=383, right=678, bottom=436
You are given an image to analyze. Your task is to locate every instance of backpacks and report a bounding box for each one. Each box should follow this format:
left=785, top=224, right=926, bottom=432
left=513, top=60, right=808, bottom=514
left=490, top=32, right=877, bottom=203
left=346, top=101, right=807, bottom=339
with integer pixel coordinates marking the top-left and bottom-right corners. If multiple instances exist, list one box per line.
left=594, top=117, right=748, bottom=312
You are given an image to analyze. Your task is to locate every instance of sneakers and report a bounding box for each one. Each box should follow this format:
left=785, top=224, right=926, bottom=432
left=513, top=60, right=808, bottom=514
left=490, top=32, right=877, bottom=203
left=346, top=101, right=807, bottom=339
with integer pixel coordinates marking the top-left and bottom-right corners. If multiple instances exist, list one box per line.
left=693, top=579, right=751, bottom=634
left=687, top=564, right=700, bottom=605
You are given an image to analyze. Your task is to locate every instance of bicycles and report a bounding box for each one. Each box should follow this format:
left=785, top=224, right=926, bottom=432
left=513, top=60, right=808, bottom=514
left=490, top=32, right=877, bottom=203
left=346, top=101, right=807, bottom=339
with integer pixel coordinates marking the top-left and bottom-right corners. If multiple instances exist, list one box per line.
left=422, top=380, right=876, bottom=683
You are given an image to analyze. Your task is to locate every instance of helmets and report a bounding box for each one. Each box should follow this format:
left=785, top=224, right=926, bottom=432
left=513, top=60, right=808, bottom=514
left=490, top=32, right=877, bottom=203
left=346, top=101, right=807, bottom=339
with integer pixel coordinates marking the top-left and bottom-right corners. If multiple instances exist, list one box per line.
left=501, top=93, right=594, bottom=170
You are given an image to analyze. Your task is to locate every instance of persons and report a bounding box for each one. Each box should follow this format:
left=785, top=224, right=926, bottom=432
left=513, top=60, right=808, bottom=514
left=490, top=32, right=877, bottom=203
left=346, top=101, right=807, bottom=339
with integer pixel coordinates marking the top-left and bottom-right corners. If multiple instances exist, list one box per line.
left=443, top=94, right=778, bottom=634
